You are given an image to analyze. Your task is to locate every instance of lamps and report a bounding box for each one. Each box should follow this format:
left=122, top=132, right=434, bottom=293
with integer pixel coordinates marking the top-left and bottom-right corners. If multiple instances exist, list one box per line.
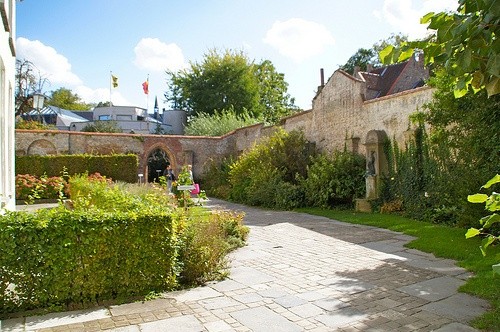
left=16, top=88, right=48, bottom=110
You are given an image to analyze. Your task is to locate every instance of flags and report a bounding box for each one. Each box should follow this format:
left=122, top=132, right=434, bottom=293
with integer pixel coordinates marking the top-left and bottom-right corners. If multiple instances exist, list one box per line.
left=142, top=81, right=149, bottom=94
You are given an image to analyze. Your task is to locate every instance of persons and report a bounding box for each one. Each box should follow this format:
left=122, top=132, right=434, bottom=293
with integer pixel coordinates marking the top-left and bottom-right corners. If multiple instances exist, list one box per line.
left=163, top=165, right=173, bottom=192
left=187, top=164, right=195, bottom=185
left=368, top=151, right=375, bottom=174
